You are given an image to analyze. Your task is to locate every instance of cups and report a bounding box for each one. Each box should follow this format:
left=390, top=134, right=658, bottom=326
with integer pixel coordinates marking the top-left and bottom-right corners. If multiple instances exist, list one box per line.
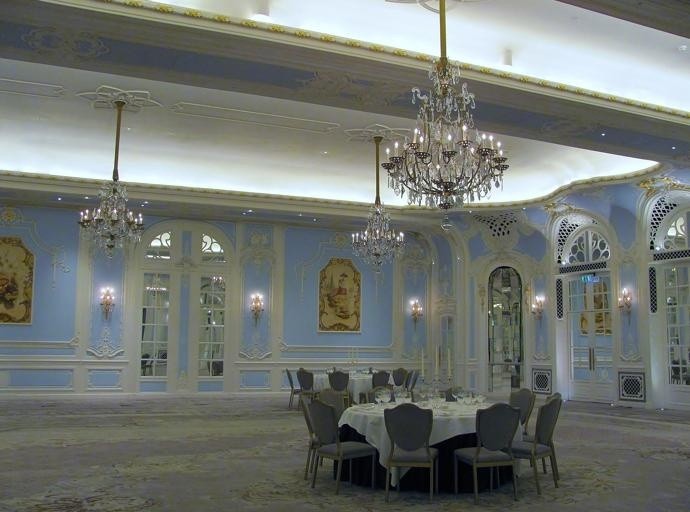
left=325, top=366, right=377, bottom=379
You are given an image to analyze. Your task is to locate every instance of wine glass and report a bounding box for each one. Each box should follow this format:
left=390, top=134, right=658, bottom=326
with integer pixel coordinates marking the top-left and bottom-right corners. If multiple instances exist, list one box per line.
left=374, top=387, right=487, bottom=415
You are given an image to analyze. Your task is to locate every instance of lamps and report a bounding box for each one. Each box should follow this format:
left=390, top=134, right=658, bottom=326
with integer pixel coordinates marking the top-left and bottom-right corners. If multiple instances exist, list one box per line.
left=349, top=136, right=406, bottom=269
left=99, top=285, right=116, bottom=319
left=250, top=293, right=264, bottom=321
left=380, top=0, right=509, bottom=218
left=531, top=294, right=544, bottom=320
left=616, top=286, right=634, bottom=314
left=410, top=297, right=424, bottom=322
left=75, top=98, right=148, bottom=256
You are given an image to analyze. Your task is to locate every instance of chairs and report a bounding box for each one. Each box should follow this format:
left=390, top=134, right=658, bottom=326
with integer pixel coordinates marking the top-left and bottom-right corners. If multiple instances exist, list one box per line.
left=282, top=366, right=566, bottom=504
